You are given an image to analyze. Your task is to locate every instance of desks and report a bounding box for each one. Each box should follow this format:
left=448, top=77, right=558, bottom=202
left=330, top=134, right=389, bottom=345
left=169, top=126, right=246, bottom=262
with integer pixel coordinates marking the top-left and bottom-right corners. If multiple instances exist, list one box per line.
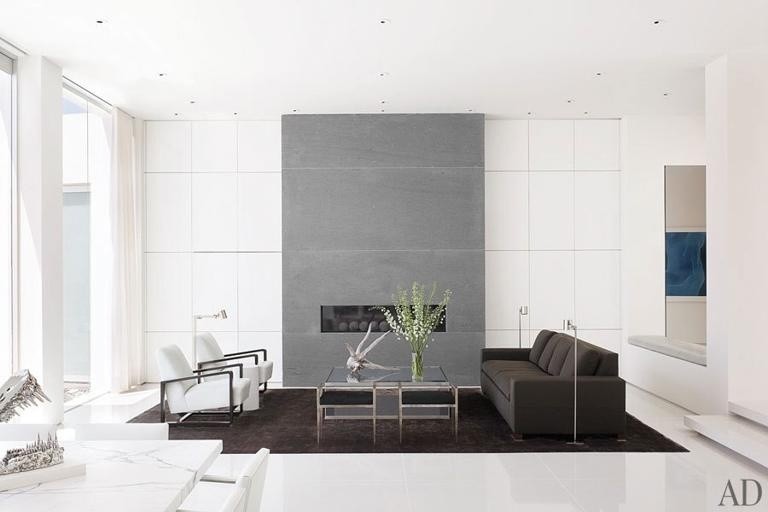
left=0, top=439, right=223, bottom=510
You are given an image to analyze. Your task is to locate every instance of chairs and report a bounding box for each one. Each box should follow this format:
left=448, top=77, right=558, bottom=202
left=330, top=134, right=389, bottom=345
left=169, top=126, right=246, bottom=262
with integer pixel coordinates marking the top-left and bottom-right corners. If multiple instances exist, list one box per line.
left=202, top=448, right=270, bottom=512
left=197, top=331, right=273, bottom=393
left=177, top=477, right=251, bottom=512
left=159, top=343, right=251, bottom=426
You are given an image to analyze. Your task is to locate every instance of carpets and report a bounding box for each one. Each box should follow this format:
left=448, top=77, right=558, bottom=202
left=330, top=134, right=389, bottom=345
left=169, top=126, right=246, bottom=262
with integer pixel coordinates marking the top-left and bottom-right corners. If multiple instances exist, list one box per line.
left=125, top=389, right=691, bottom=453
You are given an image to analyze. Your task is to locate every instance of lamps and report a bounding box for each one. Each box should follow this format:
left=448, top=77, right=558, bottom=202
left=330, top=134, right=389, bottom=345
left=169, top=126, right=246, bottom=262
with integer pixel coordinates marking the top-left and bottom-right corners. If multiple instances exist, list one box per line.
left=519, top=306, right=528, bottom=347
left=193, top=310, right=228, bottom=367
left=563, top=319, right=585, bottom=445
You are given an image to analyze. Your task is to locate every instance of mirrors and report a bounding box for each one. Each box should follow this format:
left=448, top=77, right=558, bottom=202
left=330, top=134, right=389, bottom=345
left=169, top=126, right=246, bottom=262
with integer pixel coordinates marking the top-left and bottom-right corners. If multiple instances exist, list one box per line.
left=665, top=165, right=706, bottom=344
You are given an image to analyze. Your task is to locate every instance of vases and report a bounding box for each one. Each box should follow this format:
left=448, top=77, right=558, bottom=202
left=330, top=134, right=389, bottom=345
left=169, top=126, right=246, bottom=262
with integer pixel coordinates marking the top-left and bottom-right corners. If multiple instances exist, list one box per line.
left=411, top=352, right=423, bottom=382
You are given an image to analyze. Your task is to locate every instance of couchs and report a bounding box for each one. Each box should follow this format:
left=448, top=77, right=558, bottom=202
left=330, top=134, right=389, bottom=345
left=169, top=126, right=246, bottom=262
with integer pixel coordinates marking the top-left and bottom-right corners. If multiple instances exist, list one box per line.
left=480, top=330, right=627, bottom=442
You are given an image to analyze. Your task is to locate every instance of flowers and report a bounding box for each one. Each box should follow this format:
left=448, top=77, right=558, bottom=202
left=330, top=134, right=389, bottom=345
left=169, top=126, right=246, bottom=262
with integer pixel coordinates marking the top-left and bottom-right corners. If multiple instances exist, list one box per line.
left=368, top=281, right=454, bottom=378
